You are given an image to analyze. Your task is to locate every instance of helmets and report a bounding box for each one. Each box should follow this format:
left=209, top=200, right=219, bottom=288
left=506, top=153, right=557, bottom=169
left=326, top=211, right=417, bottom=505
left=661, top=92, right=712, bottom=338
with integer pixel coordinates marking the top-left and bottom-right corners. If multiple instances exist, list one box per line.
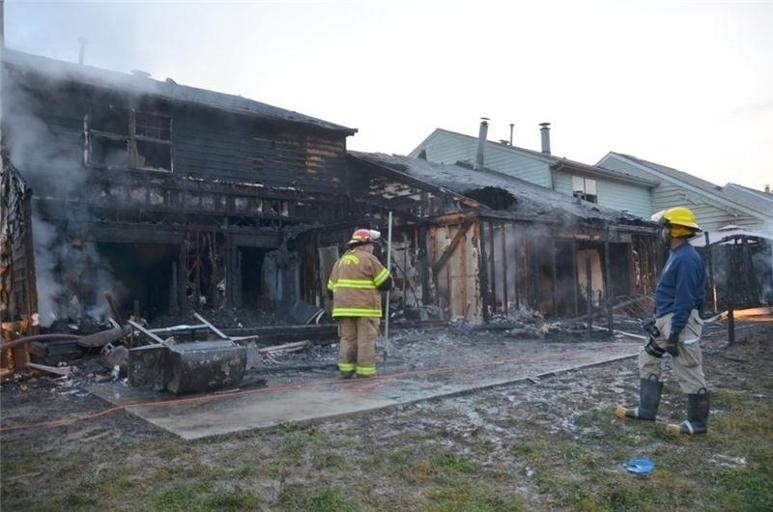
left=348, top=230, right=381, bottom=248
left=658, top=207, right=703, bottom=239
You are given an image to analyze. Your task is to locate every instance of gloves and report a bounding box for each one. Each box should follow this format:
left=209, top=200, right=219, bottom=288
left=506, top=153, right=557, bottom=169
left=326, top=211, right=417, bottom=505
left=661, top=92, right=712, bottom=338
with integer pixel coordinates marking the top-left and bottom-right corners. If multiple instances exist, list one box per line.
left=665, top=334, right=679, bottom=357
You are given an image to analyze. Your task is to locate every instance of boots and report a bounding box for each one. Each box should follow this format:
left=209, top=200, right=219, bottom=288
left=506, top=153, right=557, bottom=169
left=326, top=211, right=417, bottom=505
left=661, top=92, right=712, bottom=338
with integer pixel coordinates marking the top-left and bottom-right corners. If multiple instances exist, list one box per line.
left=666, top=393, right=708, bottom=436
left=626, top=377, right=663, bottom=421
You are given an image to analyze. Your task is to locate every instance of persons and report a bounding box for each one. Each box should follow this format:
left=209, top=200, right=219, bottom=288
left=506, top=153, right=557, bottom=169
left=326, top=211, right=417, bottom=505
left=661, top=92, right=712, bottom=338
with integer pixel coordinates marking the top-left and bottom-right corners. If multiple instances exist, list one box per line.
left=615, top=205, right=711, bottom=435
left=326, top=228, right=395, bottom=379
